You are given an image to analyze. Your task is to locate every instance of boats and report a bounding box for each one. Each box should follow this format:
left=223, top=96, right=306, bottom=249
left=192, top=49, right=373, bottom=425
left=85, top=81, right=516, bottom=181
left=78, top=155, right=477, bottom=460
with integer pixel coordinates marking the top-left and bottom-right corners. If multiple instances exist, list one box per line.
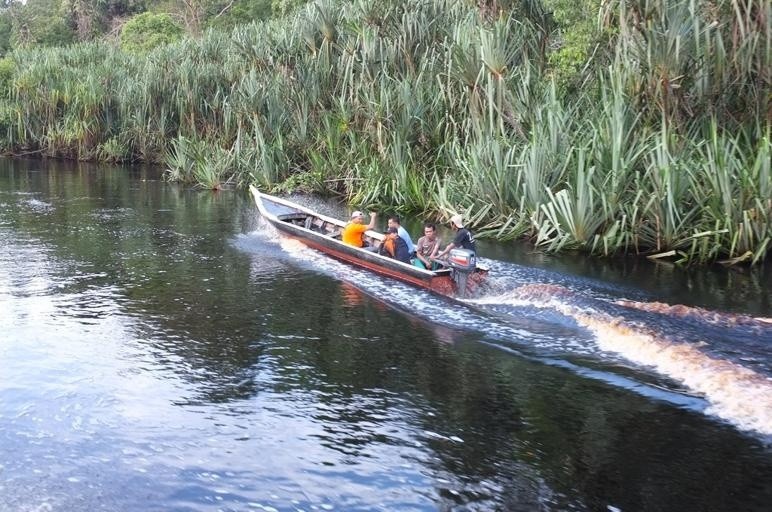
left=249, top=185, right=489, bottom=299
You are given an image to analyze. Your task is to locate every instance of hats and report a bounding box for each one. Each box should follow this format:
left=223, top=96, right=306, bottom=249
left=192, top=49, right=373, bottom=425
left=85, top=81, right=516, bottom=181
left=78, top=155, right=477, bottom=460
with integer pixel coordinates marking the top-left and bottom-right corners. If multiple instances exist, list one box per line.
left=383, top=227, right=397, bottom=234
left=451, top=215, right=464, bottom=228
left=352, top=211, right=364, bottom=218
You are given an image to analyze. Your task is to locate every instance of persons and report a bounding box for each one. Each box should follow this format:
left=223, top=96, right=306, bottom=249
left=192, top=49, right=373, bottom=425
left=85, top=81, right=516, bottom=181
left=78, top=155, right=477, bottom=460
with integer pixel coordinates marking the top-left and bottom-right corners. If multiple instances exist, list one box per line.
left=342, top=210, right=476, bottom=270
